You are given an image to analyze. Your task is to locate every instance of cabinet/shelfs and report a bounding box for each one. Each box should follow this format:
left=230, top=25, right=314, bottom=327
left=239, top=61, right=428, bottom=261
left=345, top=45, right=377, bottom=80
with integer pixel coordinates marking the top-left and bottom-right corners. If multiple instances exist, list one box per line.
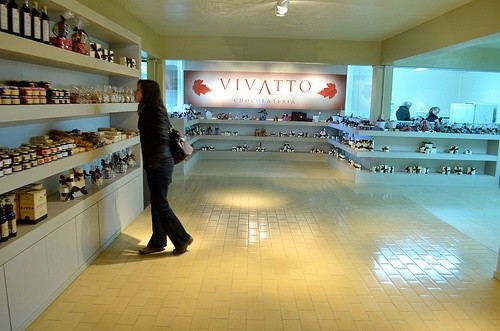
left=168, top=117, right=500, bottom=186
left=0, top=0, right=144, bottom=331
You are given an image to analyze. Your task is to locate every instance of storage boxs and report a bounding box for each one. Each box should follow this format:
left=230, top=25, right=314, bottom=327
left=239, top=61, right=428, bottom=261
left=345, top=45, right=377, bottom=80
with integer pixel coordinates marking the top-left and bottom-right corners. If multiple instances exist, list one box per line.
left=0, top=181, right=48, bottom=233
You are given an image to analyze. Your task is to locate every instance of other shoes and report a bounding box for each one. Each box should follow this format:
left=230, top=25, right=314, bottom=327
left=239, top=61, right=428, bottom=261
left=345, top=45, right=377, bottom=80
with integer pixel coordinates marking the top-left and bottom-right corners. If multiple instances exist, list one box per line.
left=173, top=234, right=193, bottom=253
left=139, top=245, right=164, bottom=254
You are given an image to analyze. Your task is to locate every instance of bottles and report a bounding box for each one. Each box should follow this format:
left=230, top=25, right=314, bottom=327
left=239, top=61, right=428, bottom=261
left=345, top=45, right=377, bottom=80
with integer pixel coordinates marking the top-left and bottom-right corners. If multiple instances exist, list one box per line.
left=0, top=198, right=18, bottom=243
left=0, top=0, right=50, bottom=44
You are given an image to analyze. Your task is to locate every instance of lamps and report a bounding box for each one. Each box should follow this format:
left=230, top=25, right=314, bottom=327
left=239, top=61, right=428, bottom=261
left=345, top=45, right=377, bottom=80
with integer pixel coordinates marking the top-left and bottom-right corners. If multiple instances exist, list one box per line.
left=274, top=0, right=290, bottom=17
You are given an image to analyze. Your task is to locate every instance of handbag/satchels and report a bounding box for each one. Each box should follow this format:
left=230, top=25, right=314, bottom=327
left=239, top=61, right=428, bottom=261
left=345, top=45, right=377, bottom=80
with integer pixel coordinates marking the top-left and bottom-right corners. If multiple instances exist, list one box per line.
left=169, top=127, right=194, bottom=164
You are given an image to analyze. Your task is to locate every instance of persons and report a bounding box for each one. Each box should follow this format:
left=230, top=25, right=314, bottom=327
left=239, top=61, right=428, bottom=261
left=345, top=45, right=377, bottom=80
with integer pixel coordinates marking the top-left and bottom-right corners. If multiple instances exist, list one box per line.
left=426, top=107, right=440, bottom=122
left=133, top=79, right=194, bottom=256
left=396, top=102, right=415, bottom=121
